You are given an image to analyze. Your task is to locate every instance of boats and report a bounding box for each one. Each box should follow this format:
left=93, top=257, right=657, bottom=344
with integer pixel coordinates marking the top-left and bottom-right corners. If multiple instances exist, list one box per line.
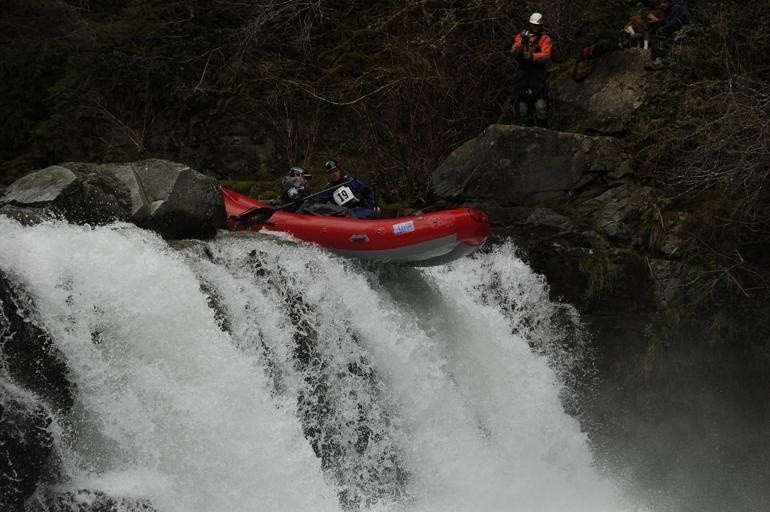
left=218, top=183, right=495, bottom=269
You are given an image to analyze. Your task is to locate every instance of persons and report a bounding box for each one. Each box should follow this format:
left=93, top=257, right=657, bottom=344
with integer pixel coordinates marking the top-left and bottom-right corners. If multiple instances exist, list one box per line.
left=645, top=0, right=689, bottom=71
left=280, top=166, right=360, bottom=219
left=616, top=1, right=652, bottom=51
left=511, top=12, right=551, bottom=128
left=310, top=159, right=381, bottom=218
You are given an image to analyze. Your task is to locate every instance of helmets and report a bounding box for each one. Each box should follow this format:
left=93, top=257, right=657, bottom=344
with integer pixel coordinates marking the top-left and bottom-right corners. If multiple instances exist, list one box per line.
left=529, top=13, right=545, bottom=24
left=288, top=167, right=312, bottom=177
left=324, top=160, right=337, bottom=173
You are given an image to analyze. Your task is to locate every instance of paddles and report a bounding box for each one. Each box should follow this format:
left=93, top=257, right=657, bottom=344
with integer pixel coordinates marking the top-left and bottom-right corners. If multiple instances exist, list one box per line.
left=239, top=179, right=355, bottom=228
